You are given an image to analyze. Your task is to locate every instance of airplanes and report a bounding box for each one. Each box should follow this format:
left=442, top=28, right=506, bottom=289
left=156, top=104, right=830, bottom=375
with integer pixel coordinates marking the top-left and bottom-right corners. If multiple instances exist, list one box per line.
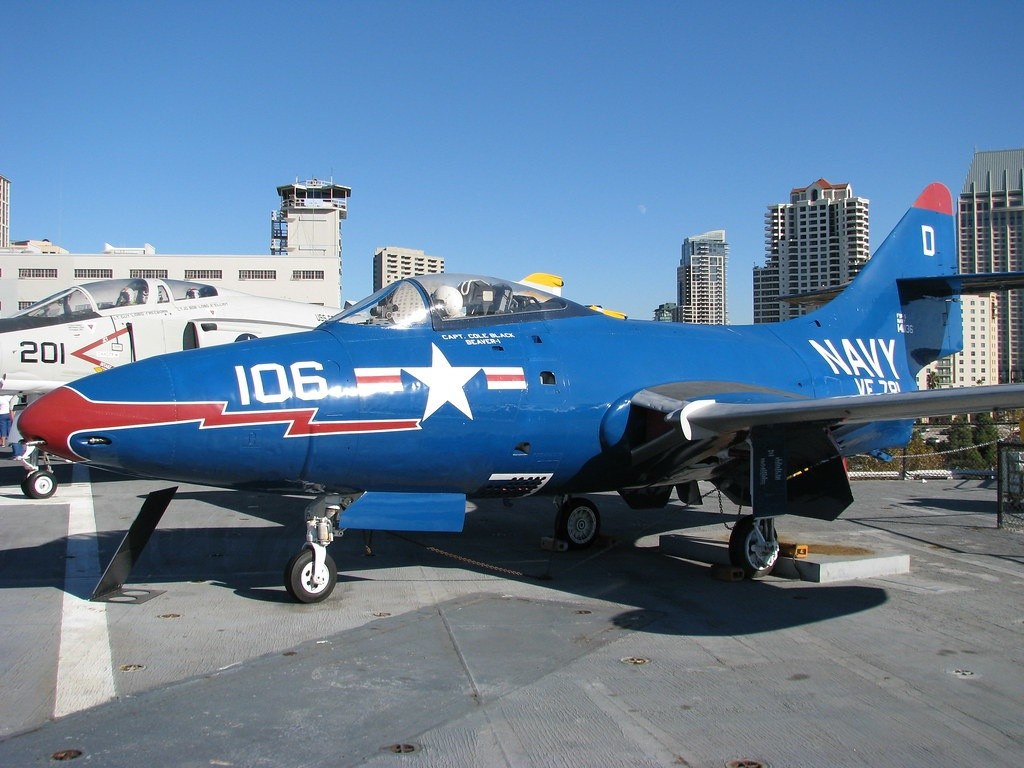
left=17, top=184, right=1024, bottom=603
left=0, top=272, right=629, bottom=500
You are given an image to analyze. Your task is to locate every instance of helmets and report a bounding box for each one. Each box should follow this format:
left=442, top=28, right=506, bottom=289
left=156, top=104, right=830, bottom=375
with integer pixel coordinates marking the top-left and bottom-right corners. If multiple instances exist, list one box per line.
left=434, top=286, right=463, bottom=315
left=186, top=288, right=199, bottom=299
left=120, top=287, right=134, bottom=301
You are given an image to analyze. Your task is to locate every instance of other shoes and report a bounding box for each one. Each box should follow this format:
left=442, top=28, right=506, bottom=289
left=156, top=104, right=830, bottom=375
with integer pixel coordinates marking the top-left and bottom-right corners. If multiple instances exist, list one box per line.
left=0, top=444, right=5, bottom=447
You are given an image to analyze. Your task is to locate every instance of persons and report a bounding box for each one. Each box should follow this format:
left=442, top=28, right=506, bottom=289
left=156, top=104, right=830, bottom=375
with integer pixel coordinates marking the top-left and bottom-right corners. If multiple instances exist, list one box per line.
left=0, top=395, right=23, bottom=460
left=433, top=285, right=463, bottom=319
left=118, top=284, right=200, bottom=304
